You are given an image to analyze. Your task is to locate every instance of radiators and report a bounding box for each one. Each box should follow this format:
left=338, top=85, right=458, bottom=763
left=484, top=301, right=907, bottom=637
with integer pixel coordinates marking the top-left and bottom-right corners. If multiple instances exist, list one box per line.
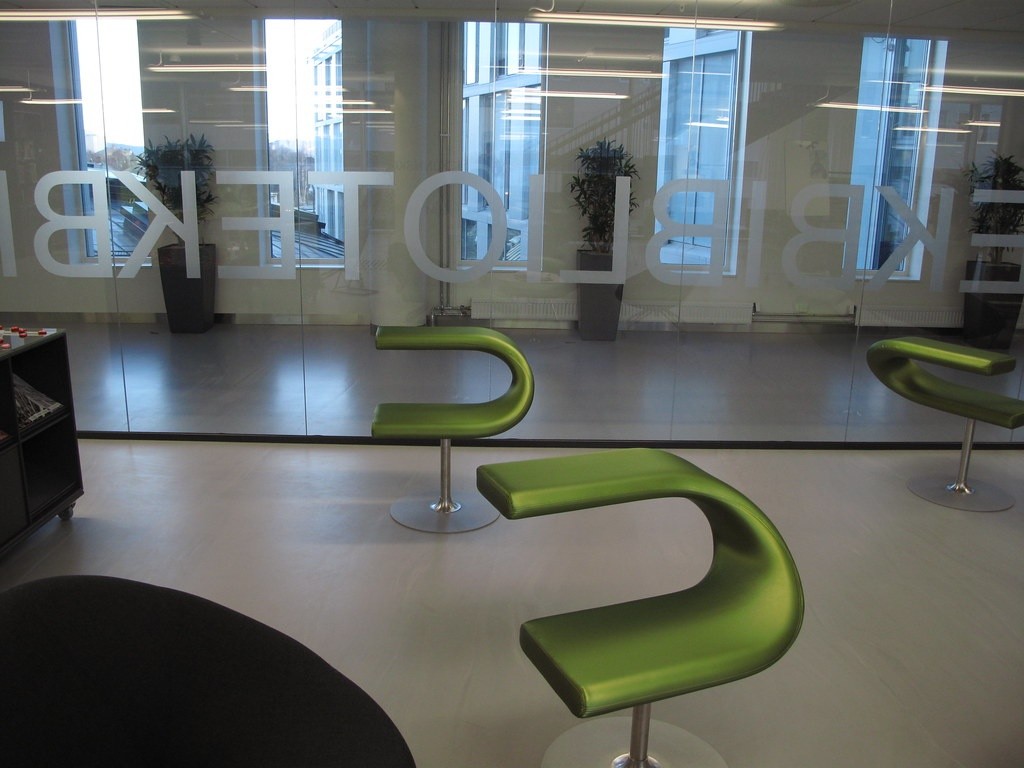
left=469, top=296, right=753, bottom=327
left=849, top=303, right=965, bottom=329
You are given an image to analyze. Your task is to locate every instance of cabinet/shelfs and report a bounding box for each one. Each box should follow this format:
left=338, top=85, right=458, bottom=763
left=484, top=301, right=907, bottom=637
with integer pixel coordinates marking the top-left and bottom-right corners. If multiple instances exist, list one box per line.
left=0, top=326, right=85, bottom=557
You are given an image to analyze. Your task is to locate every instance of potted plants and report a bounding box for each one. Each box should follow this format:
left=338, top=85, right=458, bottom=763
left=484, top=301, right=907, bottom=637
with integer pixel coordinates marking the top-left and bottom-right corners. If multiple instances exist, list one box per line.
left=132, top=133, right=217, bottom=335
left=958, top=150, right=1024, bottom=351
left=567, top=138, right=642, bottom=343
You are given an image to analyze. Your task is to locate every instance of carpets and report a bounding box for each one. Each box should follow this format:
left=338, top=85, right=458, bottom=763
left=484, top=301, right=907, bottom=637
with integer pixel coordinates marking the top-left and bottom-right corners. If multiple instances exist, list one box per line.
left=0, top=574, right=415, bottom=768
